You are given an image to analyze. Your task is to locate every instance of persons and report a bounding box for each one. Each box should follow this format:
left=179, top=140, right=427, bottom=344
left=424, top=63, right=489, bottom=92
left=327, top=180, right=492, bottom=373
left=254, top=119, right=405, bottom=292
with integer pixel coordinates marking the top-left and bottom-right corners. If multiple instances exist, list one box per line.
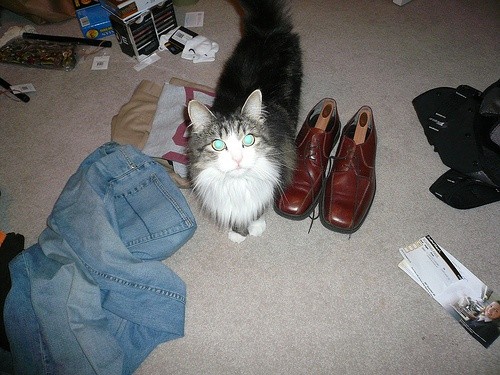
left=466, top=300, right=500, bottom=347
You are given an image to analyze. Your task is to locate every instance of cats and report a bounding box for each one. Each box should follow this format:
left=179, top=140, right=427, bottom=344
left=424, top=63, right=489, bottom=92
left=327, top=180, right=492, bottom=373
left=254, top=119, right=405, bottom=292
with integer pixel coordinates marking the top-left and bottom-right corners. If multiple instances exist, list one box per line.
left=183, top=0, right=302, bottom=244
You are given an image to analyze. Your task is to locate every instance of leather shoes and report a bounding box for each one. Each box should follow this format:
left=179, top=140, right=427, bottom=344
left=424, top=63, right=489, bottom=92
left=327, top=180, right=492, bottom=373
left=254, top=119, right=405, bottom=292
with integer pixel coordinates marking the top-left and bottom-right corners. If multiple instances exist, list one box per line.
left=273, top=98, right=340, bottom=220
left=320, top=105, right=377, bottom=234
